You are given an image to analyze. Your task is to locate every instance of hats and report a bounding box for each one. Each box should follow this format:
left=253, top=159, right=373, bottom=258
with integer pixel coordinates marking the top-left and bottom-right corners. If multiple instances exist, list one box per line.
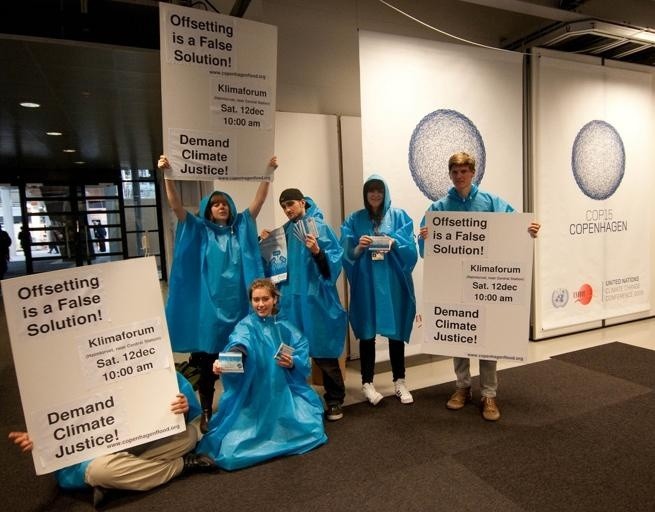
left=280, top=189, right=311, bottom=209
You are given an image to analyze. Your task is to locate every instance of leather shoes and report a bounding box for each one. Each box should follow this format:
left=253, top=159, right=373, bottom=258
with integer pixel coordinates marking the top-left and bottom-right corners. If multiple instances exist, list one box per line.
left=200, top=409, right=212, bottom=434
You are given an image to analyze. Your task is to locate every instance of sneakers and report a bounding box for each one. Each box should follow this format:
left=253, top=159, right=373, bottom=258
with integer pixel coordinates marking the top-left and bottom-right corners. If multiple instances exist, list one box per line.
left=327, top=402, right=343, bottom=420
left=93, top=487, right=107, bottom=511
left=447, top=393, right=470, bottom=409
left=183, top=452, right=216, bottom=473
left=481, top=395, right=500, bottom=421
left=394, top=379, right=414, bottom=404
left=361, top=383, right=383, bottom=406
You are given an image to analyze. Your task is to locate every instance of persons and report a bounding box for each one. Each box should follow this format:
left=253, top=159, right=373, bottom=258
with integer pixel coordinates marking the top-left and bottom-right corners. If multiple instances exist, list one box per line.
left=154, top=151, right=278, bottom=435
left=47, top=225, right=58, bottom=254
left=96, top=219, right=107, bottom=251
left=7, top=369, right=212, bottom=509
left=340, top=172, right=419, bottom=407
left=196, top=278, right=327, bottom=472
left=418, top=152, right=541, bottom=421
left=0, top=225, right=12, bottom=280
left=254, top=189, right=348, bottom=422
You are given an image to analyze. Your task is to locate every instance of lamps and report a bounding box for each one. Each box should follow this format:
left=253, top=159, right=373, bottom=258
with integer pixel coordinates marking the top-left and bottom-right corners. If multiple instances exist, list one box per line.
left=46, top=112, right=66, bottom=136
